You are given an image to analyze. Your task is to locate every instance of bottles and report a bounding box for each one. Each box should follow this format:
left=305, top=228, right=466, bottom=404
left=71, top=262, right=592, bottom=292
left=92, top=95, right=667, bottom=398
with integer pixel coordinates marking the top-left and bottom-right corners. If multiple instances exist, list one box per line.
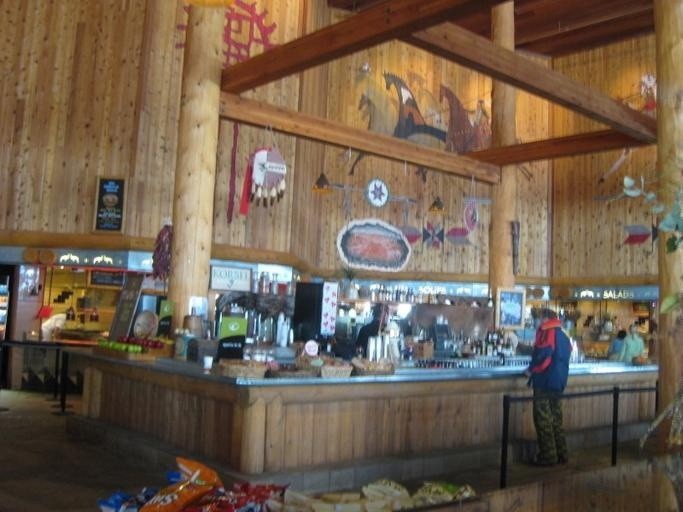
left=252, top=271, right=279, bottom=295
left=371, top=283, right=423, bottom=304
left=448, top=329, right=504, bottom=357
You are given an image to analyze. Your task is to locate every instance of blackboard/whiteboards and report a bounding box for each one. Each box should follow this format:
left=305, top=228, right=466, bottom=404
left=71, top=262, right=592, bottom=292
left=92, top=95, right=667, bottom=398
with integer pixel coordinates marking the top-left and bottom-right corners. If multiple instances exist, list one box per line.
left=107, top=271, right=147, bottom=343
left=86, top=269, right=127, bottom=291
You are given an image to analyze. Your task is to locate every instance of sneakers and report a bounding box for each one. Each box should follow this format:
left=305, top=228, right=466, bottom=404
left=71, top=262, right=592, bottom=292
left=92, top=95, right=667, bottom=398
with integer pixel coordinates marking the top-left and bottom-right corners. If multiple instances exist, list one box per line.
left=529, top=455, right=568, bottom=465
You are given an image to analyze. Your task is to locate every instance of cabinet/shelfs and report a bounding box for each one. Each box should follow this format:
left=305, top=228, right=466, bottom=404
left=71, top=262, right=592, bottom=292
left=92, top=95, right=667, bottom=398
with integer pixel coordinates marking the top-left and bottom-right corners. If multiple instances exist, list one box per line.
left=44, top=264, right=167, bottom=338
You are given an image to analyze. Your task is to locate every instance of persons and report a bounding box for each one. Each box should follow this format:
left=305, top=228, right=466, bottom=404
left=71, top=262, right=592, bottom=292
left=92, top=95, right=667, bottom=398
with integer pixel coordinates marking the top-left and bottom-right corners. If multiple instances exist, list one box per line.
left=617, top=325, right=644, bottom=365
left=355, top=303, right=389, bottom=361
left=607, top=329, right=626, bottom=360
left=523, top=307, right=572, bottom=466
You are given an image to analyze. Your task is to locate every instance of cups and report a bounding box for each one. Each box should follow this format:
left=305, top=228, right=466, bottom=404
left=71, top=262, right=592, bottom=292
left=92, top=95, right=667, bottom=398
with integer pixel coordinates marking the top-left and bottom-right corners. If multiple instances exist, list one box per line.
left=366, top=335, right=388, bottom=363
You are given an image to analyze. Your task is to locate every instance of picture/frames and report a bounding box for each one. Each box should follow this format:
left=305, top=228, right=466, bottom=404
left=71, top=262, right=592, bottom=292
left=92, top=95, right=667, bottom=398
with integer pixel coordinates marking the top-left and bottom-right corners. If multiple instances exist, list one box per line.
left=92, top=176, right=127, bottom=233
left=495, top=287, right=526, bottom=331
